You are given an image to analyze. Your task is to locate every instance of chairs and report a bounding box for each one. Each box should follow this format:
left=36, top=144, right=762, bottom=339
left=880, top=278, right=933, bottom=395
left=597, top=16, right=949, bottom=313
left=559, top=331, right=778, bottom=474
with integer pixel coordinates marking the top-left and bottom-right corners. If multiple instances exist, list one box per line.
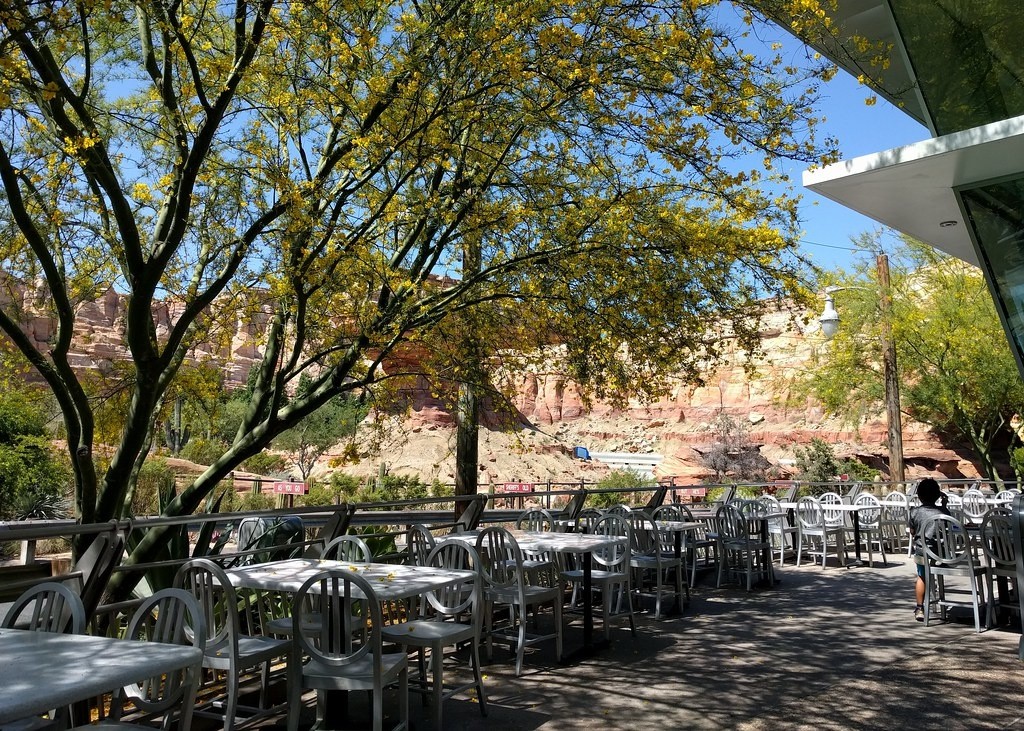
left=0, top=480, right=1024, bottom=731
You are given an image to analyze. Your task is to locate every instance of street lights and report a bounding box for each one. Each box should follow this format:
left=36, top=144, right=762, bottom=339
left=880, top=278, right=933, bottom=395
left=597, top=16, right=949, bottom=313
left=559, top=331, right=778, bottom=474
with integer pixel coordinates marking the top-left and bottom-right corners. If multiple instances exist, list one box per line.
left=816, top=253, right=906, bottom=496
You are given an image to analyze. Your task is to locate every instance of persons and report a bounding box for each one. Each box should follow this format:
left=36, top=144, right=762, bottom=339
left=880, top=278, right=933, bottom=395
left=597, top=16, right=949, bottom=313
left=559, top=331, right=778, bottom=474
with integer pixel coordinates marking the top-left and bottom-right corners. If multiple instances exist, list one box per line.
left=909, top=478, right=951, bottom=620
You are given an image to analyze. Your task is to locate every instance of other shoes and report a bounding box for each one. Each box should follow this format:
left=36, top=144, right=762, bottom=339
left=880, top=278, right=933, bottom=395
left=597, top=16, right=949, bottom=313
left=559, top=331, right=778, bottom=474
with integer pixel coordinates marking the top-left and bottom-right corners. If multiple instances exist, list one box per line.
left=932, top=603, right=952, bottom=613
left=913, top=607, right=924, bottom=619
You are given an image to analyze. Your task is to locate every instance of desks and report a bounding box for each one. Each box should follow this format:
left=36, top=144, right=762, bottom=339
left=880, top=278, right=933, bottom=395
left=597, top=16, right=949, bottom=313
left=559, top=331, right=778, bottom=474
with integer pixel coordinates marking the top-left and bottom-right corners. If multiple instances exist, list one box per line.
left=941, top=524, right=1014, bottom=629
left=766, top=502, right=886, bottom=569
left=196, top=558, right=479, bottom=731
left=542, top=518, right=705, bottom=619
left=0, top=627, right=203, bottom=731
left=411, top=530, right=628, bottom=666
left=661, top=512, right=788, bottom=593
left=877, top=498, right=1012, bottom=557
